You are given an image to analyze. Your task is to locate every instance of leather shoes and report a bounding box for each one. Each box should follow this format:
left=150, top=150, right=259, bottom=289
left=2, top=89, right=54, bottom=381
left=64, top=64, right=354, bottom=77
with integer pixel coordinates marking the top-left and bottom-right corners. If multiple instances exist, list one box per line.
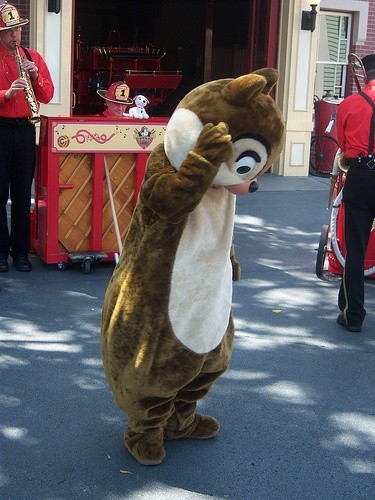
left=0, top=257, right=9, bottom=272
left=13, top=256, right=32, bottom=272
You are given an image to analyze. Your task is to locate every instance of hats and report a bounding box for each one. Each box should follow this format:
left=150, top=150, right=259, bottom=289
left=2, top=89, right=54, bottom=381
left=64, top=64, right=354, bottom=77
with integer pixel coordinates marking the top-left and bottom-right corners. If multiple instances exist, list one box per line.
left=361, top=54, right=375, bottom=72
left=97, top=81, right=133, bottom=105
left=0, top=1, right=29, bottom=31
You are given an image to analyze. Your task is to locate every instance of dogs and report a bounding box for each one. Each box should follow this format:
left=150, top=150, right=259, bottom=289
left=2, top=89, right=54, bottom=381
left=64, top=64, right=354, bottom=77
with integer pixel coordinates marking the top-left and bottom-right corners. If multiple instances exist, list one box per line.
left=123, top=94, right=152, bottom=120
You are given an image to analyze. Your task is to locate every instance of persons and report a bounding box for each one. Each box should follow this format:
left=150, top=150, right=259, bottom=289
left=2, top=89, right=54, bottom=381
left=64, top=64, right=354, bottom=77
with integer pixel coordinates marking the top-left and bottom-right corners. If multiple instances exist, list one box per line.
left=336, top=54, right=375, bottom=333
left=96, top=81, right=133, bottom=117
left=0, top=1, right=55, bottom=272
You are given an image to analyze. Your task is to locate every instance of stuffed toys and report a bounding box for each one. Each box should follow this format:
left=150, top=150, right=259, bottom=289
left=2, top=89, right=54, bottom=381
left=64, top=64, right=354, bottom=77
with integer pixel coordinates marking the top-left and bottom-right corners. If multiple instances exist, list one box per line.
left=123, top=95, right=149, bottom=120
left=102, top=68, right=285, bottom=465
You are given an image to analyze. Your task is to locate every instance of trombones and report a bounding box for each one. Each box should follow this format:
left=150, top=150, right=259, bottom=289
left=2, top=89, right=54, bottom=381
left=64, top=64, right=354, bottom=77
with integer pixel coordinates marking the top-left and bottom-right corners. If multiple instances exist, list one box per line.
left=347, top=52, right=368, bottom=91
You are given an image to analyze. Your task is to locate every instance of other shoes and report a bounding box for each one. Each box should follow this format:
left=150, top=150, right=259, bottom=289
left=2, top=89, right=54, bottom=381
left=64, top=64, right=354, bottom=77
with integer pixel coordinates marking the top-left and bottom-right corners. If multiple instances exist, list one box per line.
left=337, top=312, right=361, bottom=332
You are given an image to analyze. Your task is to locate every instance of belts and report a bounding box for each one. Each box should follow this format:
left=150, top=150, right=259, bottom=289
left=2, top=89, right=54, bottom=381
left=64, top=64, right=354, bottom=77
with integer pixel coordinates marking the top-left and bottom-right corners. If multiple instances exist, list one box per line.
left=350, top=161, right=375, bottom=168
left=0, top=118, right=31, bottom=128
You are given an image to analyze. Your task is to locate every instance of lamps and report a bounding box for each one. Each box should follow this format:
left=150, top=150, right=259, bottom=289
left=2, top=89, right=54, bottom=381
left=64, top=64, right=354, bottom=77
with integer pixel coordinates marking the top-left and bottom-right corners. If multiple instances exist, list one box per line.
left=48, top=0, right=60, bottom=14
left=300, top=0, right=321, bottom=32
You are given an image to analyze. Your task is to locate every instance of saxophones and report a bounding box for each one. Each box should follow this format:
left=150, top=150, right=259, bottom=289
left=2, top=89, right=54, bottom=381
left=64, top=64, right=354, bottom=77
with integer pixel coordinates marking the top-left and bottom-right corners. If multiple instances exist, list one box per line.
left=13, top=39, right=43, bottom=124
